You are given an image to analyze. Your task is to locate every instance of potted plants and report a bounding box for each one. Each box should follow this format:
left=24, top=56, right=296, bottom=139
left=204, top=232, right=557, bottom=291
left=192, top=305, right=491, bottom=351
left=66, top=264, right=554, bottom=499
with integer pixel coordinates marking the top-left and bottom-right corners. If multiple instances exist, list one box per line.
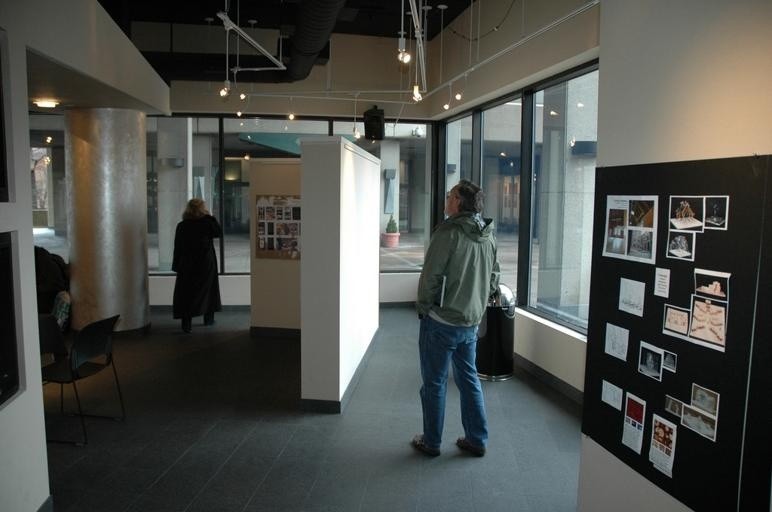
left=381, top=215, right=401, bottom=248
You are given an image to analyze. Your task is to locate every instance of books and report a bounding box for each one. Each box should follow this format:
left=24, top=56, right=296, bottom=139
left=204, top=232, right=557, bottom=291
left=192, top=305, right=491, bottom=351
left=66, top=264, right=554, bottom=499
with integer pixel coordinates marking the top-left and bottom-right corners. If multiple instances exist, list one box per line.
left=432, top=275, right=448, bottom=309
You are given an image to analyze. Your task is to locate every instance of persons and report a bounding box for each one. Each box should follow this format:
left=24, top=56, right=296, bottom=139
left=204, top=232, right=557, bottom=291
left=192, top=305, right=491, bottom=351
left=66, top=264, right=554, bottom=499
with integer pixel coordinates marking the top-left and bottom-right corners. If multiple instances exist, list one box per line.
left=170, top=197, right=222, bottom=333
left=34, top=240, right=67, bottom=313
left=410, top=180, right=502, bottom=459
left=675, top=200, right=695, bottom=221
left=642, top=353, right=661, bottom=380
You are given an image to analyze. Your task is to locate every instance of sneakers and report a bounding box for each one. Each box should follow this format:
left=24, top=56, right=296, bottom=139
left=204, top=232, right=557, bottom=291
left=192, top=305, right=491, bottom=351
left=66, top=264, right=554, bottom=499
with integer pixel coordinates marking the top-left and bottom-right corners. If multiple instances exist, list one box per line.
left=456, top=437, right=485, bottom=457
left=413, top=434, right=441, bottom=457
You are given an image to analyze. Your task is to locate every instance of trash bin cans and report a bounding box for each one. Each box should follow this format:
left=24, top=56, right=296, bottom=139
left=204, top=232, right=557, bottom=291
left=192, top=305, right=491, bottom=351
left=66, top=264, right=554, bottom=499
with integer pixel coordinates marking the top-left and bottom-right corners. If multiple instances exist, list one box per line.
left=475, top=283, right=516, bottom=382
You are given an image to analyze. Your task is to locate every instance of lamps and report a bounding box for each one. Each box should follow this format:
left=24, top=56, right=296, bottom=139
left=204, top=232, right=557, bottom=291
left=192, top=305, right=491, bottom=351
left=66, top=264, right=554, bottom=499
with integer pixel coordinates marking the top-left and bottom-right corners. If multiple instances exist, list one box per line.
left=218, top=1, right=250, bottom=116
left=397, top=1, right=465, bottom=109
left=287, top=97, right=295, bottom=120
left=352, top=101, right=360, bottom=139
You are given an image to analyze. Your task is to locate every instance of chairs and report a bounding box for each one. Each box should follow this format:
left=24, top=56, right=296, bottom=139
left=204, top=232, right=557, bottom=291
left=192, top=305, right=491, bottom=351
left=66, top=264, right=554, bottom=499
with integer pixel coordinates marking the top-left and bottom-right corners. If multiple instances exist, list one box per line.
left=39, top=311, right=127, bottom=445
